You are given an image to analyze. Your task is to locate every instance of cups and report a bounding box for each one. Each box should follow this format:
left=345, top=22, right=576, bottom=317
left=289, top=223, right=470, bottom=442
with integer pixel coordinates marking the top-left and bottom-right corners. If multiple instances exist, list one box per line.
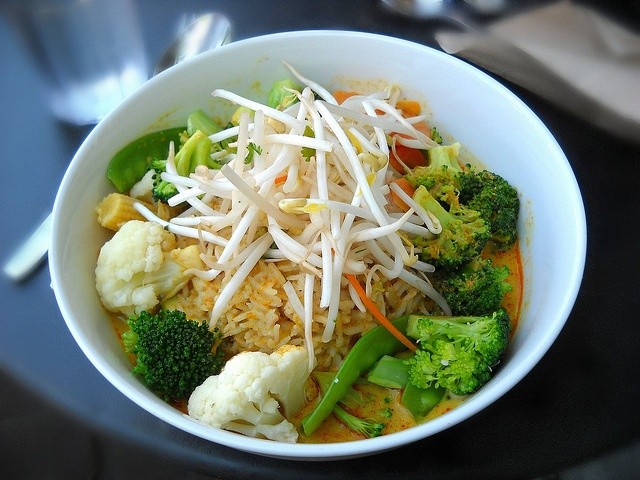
left=16, top=2, right=148, bottom=127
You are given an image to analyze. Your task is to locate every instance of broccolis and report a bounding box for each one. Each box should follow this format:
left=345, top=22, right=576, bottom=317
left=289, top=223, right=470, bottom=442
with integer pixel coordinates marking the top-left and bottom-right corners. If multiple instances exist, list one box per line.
left=122, top=308, right=234, bottom=400
left=187, top=344, right=310, bottom=446
left=439, top=257, right=512, bottom=316
left=414, top=183, right=491, bottom=258
left=408, top=143, right=484, bottom=205
left=91, top=219, right=187, bottom=319
left=409, top=308, right=513, bottom=395
left=464, top=170, right=520, bottom=250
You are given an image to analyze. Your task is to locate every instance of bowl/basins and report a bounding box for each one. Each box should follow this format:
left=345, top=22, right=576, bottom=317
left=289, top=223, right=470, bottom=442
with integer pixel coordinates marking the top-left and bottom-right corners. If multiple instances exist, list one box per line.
left=48, top=28, right=587, bottom=463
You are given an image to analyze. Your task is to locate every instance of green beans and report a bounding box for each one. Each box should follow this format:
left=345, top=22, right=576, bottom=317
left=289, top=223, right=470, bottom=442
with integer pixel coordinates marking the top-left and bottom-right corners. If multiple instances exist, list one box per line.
left=105, top=127, right=189, bottom=192
left=303, top=318, right=405, bottom=434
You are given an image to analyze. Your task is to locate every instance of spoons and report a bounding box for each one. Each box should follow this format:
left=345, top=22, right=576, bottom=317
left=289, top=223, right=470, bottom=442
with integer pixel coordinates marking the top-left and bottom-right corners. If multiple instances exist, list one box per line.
left=3, top=13, right=234, bottom=283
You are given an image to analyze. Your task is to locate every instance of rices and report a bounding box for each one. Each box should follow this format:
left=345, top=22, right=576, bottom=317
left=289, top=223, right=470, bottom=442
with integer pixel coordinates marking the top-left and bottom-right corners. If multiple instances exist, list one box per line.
left=165, top=196, right=426, bottom=372
